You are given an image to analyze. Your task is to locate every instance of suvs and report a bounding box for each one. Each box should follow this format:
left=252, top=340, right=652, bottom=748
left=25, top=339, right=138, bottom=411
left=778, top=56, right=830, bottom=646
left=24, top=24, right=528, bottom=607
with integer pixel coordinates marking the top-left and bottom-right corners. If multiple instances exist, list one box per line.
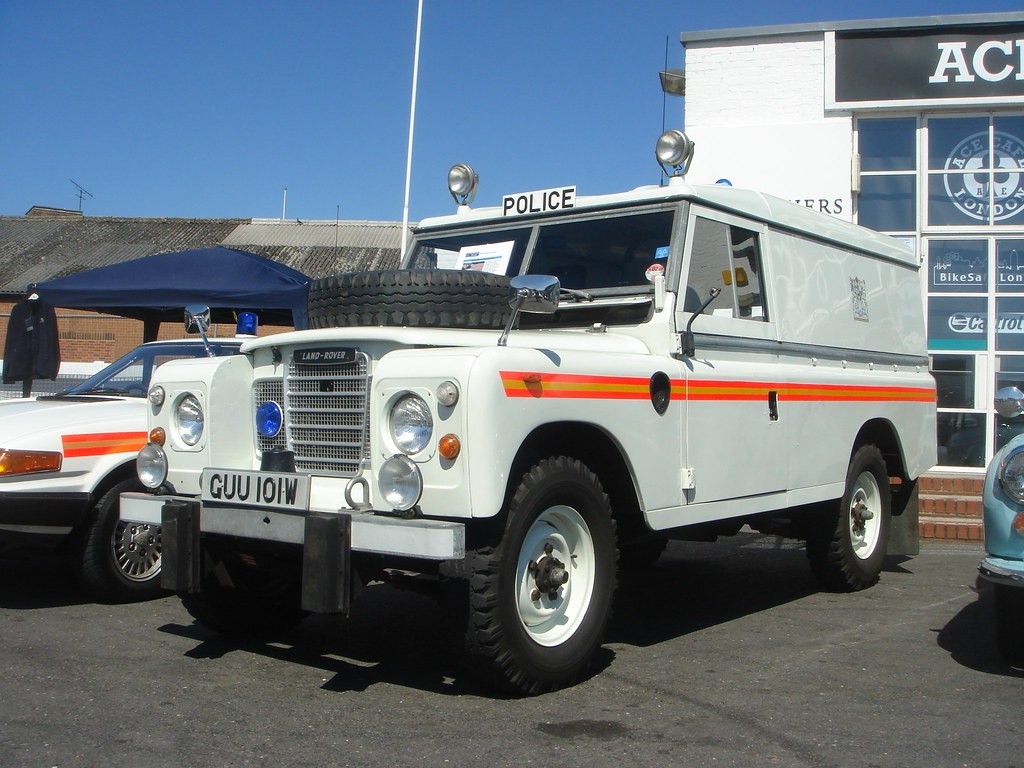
left=120, top=129, right=942, bottom=705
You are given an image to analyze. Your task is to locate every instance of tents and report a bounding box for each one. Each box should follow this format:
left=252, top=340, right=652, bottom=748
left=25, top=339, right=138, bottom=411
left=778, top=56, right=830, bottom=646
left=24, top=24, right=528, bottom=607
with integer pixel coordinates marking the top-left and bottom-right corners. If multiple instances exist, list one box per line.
left=22, top=248, right=314, bottom=398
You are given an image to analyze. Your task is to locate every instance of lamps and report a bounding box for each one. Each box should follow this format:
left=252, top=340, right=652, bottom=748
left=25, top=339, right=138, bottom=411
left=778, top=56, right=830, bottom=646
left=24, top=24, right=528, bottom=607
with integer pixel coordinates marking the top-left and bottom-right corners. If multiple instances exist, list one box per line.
left=659, top=68, right=685, bottom=95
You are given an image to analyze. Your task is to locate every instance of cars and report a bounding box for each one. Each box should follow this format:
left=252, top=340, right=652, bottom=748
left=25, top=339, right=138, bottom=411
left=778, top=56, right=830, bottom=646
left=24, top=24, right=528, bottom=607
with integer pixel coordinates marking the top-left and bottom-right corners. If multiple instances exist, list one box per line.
left=0, top=312, right=268, bottom=606
left=971, top=383, right=1024, bottom=592
